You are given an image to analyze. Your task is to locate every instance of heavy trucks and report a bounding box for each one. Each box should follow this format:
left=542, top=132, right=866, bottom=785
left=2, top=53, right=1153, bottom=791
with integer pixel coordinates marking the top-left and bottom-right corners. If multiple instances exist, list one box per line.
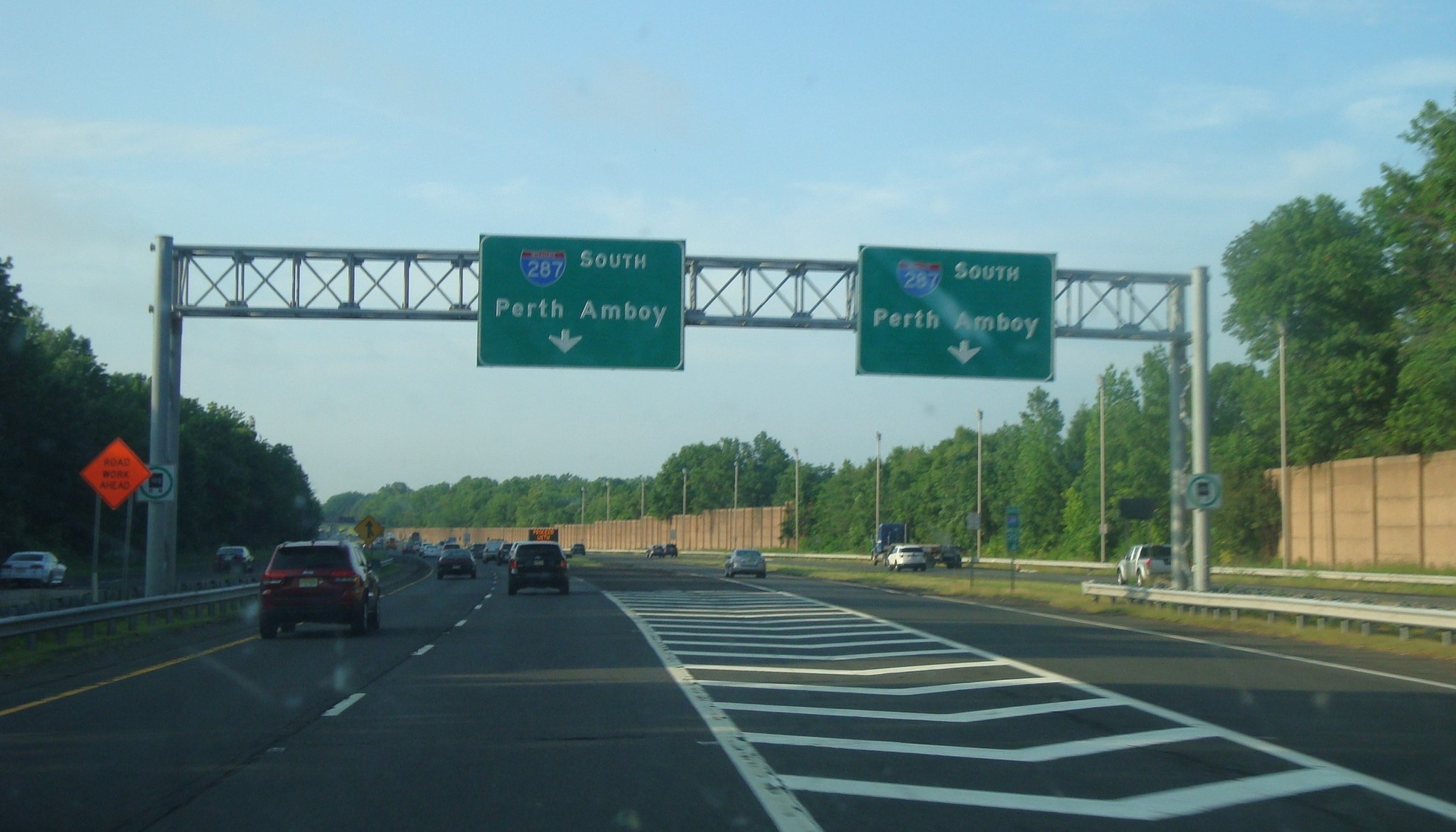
left=869, top=521, right=967, bottom=569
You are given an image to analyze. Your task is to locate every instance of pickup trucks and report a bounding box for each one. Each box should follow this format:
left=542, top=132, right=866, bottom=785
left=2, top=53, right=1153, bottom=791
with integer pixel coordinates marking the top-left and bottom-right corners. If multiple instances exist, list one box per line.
left=1116, top=542, right=1193, bottom=587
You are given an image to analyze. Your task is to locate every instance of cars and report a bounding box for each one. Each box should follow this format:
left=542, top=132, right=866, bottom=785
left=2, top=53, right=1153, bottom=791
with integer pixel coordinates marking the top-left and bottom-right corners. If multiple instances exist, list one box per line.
left=363, top=531, right=520, bottom=566
left=504, top=541, right=573, bottom=596
left=886, top=545, right=927, bottom=573
left=1, top=550, right=69, bottom=589
left=570, top=544, right=585, bottom=556
left=258, top=537, right=381, bottom=641
left=436, top=549, right=478, bottom=579
left=724, top=550, right=766, bottom=579
left=213, top=546, right=255, bottom=574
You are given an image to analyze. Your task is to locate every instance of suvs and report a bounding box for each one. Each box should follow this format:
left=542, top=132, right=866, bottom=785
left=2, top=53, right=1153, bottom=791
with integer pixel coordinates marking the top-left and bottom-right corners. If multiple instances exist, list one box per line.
left=661, top=544, right=678, bottom=557
left=645, top=545, right=665, bottom=558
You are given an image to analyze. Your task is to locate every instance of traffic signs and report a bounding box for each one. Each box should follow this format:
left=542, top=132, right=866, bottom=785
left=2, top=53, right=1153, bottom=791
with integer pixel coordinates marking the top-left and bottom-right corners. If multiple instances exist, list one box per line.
left=472, top=233, right=688, bottom=370
left=854, top=242, right=1061, bottom=383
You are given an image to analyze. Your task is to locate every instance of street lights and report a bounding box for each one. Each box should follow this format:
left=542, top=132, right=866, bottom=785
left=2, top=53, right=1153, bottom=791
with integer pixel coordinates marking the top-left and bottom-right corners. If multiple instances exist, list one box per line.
left=733, top=461, right=739, bottom=550
left=1097, top=374, right=1108, bottom=563
left=875, top=431, right=882, bottom=542
left=793, top=447, right=801, bottom=553
left=605, top=481, right=611, bottom=521
left=681, top=468, right=687, bottom=515
left=580, top=486, right=585, bottom=525
left=639, top=476, right=645, bottom=516
left=974, top=409, right=983, bottom=559
